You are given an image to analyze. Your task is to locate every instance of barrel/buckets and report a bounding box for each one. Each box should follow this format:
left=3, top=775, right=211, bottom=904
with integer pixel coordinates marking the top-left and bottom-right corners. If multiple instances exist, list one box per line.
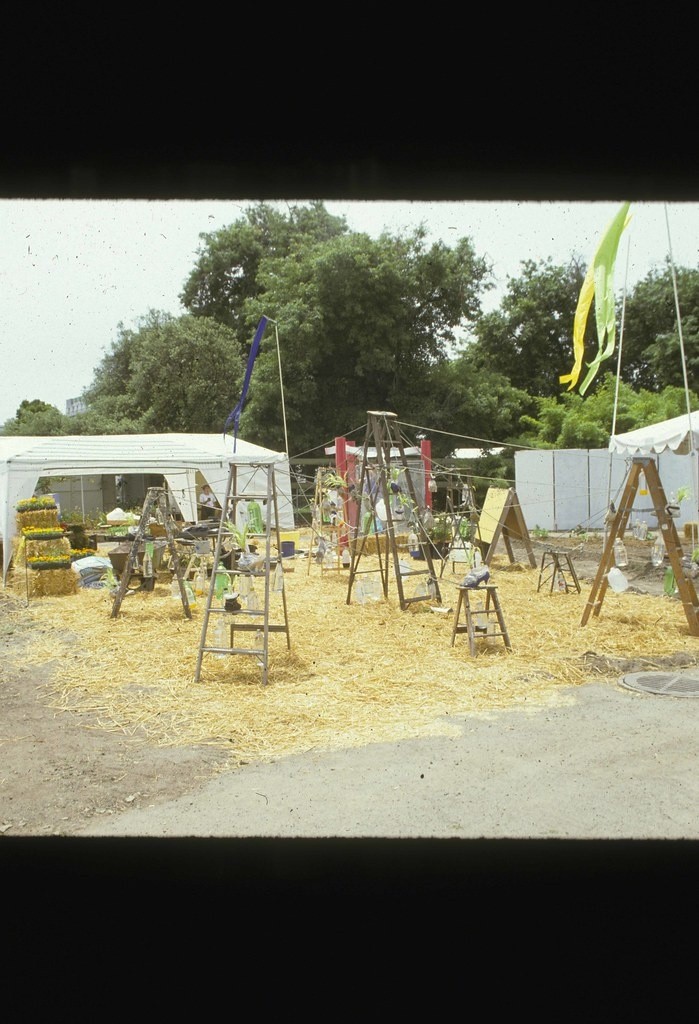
left=281, top=541, right=295, bottom=557
left=280, top=532, right=300, bottom=550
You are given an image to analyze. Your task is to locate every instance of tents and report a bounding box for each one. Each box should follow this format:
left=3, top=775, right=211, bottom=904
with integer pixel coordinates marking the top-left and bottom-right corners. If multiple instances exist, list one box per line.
left=0, top=433, right=294, bottom=588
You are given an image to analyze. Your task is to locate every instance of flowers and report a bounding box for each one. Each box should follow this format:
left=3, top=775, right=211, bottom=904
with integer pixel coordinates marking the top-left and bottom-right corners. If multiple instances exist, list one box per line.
left=22, top=526, right=65, bottom=535
left=12, top=496, right=56, bottom=510
left=27, top=556, right=71, bottom=563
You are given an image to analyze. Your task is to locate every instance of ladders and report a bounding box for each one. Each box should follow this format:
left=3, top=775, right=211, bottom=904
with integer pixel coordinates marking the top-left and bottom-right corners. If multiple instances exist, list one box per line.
left=536, top=549, right=582, bottom=597
left=307, top=468, right=353, bottom=581
left=346, top=409, right=442, bottom=610
left=439, top=468, right=486, bottom=578
left=194, top=460, right=292, bottom=685
left=451, top=583, right=512, bottom=657
left=578, top=456, right=698, bottom=635
left=109, top=486, right=194, bottom=621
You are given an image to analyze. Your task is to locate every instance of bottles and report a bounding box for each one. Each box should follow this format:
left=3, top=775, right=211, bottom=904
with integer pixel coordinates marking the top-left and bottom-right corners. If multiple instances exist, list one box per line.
left=394, top=557, right=410, bottom=581
left=213, top=619, right=227, bottom=658
left=651, top=534, right=665, bottom=567
left=607, top=567, right=628, bottom=593
left=663, top=565, right=676, bottom=597
left=238, top=575, right=260, bottom=619
left=214, top=563, right=232, bottom=603
left=247, top=499, right=263, bottom=535
left=487, top=618, right=499, bottom=644
left=171, top=574, right=181, bottom=599
left=408, top=533, right=419, bottom=552
left=271, top=559, right=284, bottom=594
left=613, top=537, right=628, bottom=567
left=363, top=512, right=382, bottom=533
left=254, top=629, right=265, bottom=666
left=342, top=547, right=350, bottom=568
left=476, top=601, right=488, bottom=630
left=155, top=507, right=164, bottom=527
left=633, top=519, right=648, bottom=540
left=181, top=572, right=204, bottom=610
left=143, top=550, right=154, bottom=578
left=325, top=549, right=333, bottom=568
left=554, top=573, right=565, bottom=592
left=355, top=574, right=382, bottom=603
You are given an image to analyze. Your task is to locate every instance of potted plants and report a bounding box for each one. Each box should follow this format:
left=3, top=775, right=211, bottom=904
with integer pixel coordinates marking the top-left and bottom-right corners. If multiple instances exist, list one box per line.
left=219, top=519, right=260, bottom=570
left=222, top=572, right=242, bottom=611
left=665, top=484, right=691, bottom=515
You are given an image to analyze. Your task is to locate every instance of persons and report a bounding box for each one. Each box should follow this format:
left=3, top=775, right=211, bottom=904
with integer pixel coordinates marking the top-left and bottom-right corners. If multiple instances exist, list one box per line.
left=199, top=484, right=217, bottom=520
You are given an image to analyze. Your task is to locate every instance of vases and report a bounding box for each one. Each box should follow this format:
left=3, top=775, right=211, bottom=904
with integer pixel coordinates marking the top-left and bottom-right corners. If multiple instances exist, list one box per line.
left=18, top=506, right=57, bottom=513
left=31, top=563, right=71, bottom=569
left=27, top=535, right=64, bottom=540
